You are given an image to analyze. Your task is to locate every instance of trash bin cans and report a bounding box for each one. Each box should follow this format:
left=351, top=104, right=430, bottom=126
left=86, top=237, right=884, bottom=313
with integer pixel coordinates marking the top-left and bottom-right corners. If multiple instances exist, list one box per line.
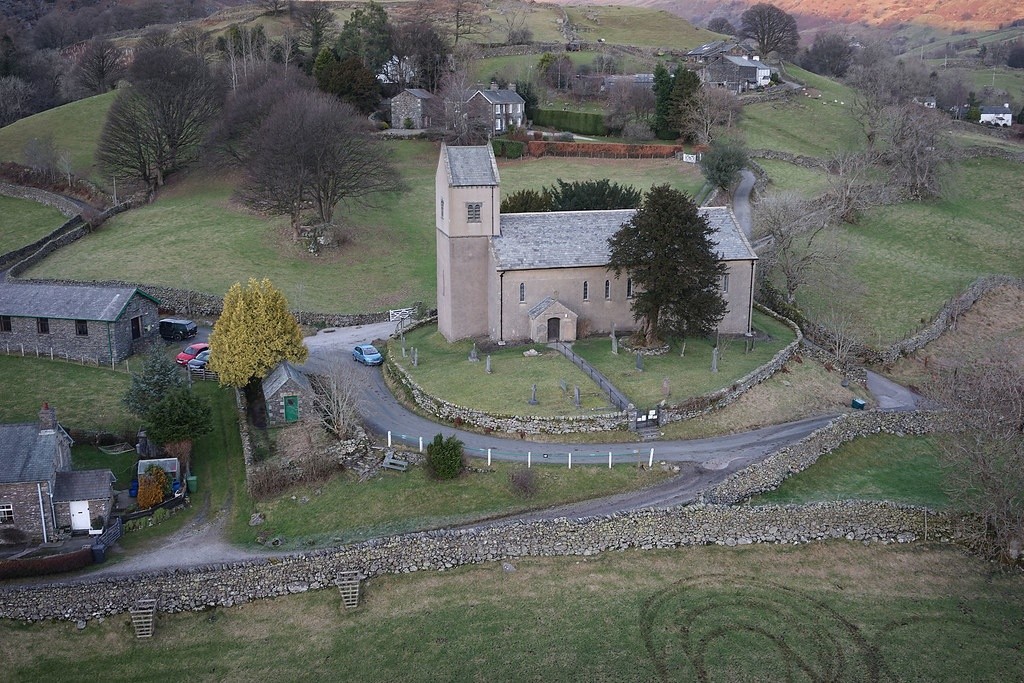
left=852, top=398, right=865, bottom=410
left=91, top=543, right=108, bottom=564
left=187, top=475, right=199, bottom=493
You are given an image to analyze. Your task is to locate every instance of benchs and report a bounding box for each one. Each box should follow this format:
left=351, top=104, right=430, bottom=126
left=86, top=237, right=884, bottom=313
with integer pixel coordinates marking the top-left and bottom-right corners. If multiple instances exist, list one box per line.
left=382, top=459, right=408, bottom=473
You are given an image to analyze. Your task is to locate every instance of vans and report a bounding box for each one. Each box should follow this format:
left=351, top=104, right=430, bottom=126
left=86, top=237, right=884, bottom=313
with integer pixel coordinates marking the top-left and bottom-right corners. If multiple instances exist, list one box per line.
left=158, top=319, right=197, bottom=341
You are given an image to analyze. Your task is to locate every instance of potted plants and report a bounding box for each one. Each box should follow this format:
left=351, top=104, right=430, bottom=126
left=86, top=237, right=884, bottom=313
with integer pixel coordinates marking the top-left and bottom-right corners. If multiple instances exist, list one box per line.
left=89, top=515, right=104, bottom=535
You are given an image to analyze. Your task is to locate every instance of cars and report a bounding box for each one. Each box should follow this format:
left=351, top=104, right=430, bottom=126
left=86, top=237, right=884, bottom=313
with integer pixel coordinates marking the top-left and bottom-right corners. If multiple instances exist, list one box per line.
left=175, top=343, right=210, bottom=365
left=186, top=350, right=212, bottom=373
left=352, top=343, right=383, bottom=366
